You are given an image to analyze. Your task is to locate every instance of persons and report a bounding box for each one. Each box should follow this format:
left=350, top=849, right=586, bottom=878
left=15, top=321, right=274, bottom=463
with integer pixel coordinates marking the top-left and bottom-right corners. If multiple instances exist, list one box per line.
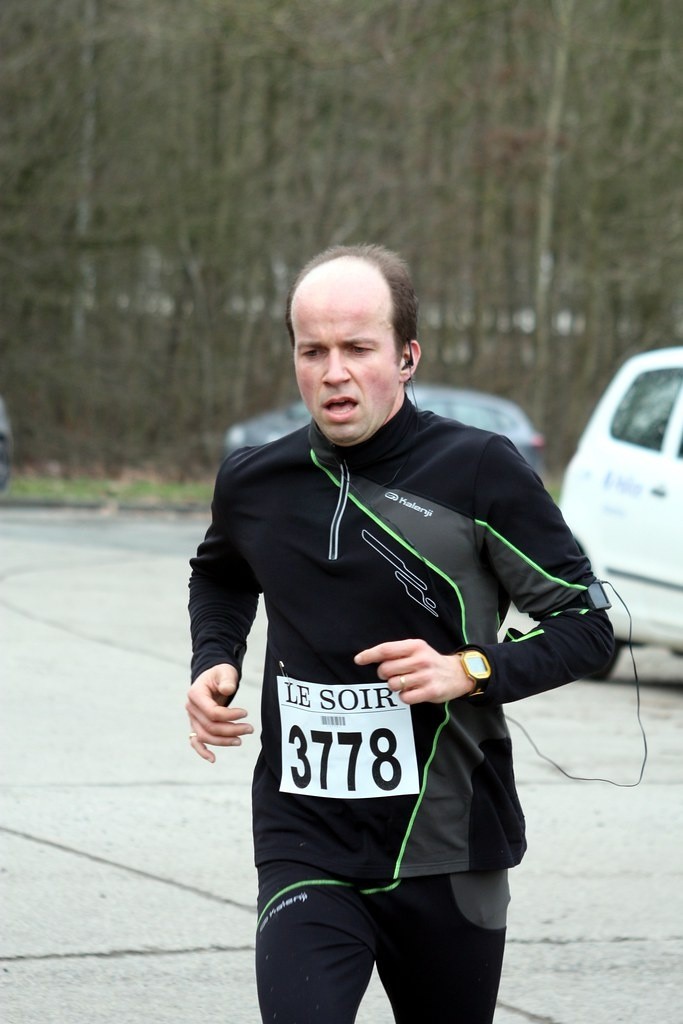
left=186, top=244, right=619, bottom=1024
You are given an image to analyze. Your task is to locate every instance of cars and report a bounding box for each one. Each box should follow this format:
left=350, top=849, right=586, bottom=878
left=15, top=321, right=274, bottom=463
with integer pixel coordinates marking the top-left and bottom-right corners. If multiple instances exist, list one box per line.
left=223, top=386, right=551, bottom=489
left=551, top=346, right=683, bottom=658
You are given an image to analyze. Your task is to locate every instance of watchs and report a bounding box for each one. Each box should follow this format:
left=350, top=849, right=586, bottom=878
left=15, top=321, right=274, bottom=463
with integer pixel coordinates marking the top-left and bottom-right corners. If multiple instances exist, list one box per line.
left=455, top=649, right=491, bottom=699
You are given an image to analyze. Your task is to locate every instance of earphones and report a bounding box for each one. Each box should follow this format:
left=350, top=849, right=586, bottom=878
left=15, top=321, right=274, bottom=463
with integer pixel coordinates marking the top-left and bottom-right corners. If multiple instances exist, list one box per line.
left=401, top=356, right=413, bottom=370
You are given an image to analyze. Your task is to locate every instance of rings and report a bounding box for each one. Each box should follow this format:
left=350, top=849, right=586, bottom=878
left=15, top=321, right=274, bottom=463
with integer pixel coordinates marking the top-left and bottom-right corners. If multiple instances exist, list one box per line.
left=399, top=675, right=407, bottom=693
left=188, top=733, right=198, bottom=739
